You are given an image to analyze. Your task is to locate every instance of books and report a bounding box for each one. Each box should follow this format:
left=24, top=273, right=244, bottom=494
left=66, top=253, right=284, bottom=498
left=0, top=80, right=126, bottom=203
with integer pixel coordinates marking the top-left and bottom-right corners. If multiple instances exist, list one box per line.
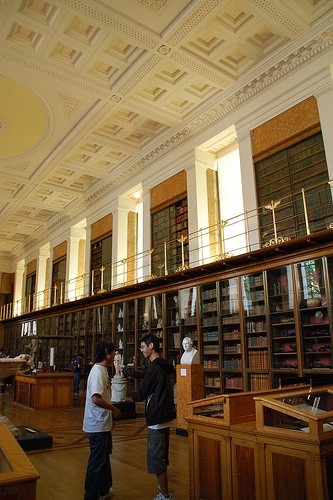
left=1, top=312, right=70, bottom=367
left=180, top=255, right=332, bottom=400
left=71, top=305, right=113, bottom=364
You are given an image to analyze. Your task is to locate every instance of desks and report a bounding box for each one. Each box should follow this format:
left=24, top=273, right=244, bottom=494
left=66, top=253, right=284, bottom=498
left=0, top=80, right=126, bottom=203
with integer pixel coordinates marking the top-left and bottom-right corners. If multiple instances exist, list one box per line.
left=185, top=383, right=333, bottom=500
left=14, top=371, right=75, bottom=408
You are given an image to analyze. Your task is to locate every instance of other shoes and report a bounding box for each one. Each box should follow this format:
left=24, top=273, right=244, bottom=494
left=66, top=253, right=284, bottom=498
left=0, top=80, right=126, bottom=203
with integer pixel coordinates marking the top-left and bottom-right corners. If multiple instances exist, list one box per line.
left=99, top=487, right=114, bottom=500
left=153, top=490, right=173, bottom=500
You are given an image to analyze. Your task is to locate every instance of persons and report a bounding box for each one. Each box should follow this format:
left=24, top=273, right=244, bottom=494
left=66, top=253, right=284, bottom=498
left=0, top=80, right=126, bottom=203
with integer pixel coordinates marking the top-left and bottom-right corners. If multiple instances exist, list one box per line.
left=114, top=351, right=125, bottom=379
left=73, top=353, right=84, bottom=396
left=180, top=337, right=200, bottom=365
left=132, top=333, right=176, bottom=500
left=82, top=341, right=122, bottom=500
left=0, top=348, right=7, bottom=357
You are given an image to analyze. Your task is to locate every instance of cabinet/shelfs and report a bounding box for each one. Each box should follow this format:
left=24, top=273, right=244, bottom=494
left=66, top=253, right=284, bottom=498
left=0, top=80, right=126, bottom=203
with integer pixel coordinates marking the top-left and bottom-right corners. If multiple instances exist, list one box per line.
left=0, top=248, right=333, bottom=404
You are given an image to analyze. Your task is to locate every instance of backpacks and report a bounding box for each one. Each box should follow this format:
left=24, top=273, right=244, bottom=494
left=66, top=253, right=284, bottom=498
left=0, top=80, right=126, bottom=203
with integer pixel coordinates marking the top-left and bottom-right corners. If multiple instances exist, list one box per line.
left=70, top=358, right=79, bottom=373
